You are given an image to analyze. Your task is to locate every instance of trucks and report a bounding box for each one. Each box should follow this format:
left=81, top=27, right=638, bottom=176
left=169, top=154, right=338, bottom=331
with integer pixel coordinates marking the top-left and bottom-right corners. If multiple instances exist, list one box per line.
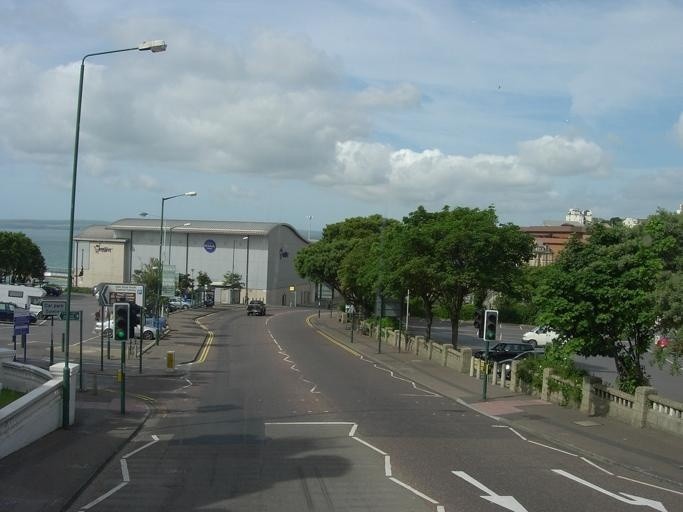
left=0, top=284, right=49, bottom=320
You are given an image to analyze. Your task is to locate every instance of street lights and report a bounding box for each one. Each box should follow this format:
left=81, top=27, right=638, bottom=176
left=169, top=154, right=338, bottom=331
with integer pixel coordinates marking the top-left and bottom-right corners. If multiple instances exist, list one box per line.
left=61, top=40, right=168, bottom=432
left=168, top=223, right=193, bottom=265
left=157, top=192, right=199, bottom=316
left=242, top=236, right=250, bottom=307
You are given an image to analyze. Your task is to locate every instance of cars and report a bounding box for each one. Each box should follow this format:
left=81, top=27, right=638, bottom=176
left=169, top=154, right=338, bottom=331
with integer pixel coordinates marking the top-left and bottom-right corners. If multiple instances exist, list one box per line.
left=41, top=284, right=62, bottom=296
left=95, top=319, right=159, bottom=340
left=169, top=297, right=191, bottom=310
left=522, top=326, right=573, bottom=349
left=0, top=302, right=37, bottom=324
left=247, top=300, right=267, bottom=317
left=474, top=342, right=535, bottom=363
left=498, top=351, right=548, bottom=381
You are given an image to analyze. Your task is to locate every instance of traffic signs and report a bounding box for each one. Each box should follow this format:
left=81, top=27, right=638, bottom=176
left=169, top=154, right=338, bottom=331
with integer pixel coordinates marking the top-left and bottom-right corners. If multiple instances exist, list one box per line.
left=12, top=307, right=29, bottom=335
left=58, top=312, right=79, bottom=320
left=41, top=300, right=66, bottom=317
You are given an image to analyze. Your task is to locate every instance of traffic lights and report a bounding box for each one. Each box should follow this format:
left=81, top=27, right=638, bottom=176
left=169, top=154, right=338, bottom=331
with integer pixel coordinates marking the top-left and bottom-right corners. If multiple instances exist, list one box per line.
left=113, top=302, right=128, bottom=340
left=473, top=309, right=483, bottom=338
left=483, top=310, right=500, bottom=342
left=129, top=302, right=142, bottom=338
